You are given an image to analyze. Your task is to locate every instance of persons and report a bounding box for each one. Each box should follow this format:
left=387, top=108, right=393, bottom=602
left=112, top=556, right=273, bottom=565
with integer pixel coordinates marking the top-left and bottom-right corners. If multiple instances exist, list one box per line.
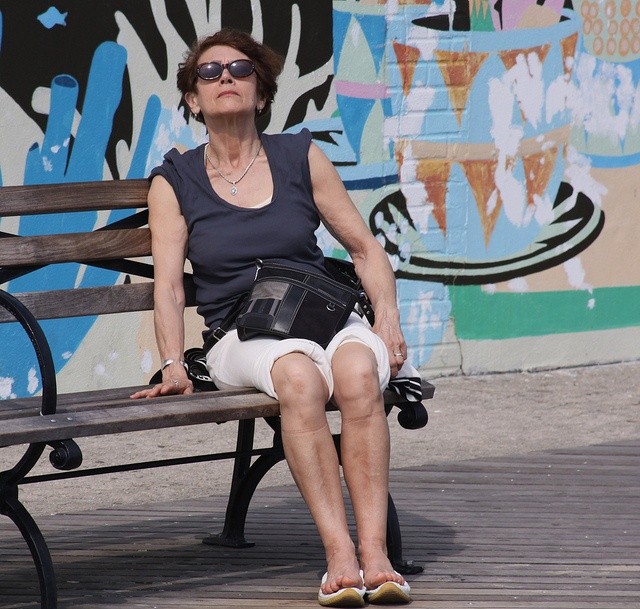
left=130, top=31, right=408, bottom=594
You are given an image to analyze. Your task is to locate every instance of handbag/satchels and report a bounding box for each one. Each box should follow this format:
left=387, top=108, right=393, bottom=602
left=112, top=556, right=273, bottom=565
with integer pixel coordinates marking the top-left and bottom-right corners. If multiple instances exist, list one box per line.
left=235, top=257, right=364, bottom=350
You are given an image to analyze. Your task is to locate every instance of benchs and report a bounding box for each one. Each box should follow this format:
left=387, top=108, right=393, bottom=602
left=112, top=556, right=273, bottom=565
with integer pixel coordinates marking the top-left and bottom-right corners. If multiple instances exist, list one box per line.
left=1, top=178, right=435, bottom=606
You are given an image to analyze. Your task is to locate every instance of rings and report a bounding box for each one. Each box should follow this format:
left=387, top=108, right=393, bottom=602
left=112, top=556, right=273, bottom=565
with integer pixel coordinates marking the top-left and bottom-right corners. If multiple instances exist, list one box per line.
left=171, top=379, right=180, bottom=386
left=394, top=353, right=403, bottom=356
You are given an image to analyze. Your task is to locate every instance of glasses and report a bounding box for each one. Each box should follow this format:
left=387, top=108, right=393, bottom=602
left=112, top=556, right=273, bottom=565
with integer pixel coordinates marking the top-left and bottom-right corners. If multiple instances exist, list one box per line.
left=196, top=59, right=256, bottom=80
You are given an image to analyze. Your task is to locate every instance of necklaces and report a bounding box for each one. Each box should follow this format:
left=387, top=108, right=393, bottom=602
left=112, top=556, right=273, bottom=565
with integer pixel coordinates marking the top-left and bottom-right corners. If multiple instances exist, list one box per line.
left=205, top=142, right=262, bottom=196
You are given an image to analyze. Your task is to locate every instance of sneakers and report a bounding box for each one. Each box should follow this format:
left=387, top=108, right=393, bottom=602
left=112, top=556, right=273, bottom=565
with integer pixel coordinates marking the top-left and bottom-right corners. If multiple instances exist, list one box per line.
left=365, top=570, right=410, bottom=605
left=318, top=570, right=366, bottom=607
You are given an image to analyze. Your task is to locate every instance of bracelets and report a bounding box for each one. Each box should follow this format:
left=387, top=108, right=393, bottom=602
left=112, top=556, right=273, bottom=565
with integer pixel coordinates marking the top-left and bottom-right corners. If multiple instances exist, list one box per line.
left=160, top=358, right=189, bottom=373
